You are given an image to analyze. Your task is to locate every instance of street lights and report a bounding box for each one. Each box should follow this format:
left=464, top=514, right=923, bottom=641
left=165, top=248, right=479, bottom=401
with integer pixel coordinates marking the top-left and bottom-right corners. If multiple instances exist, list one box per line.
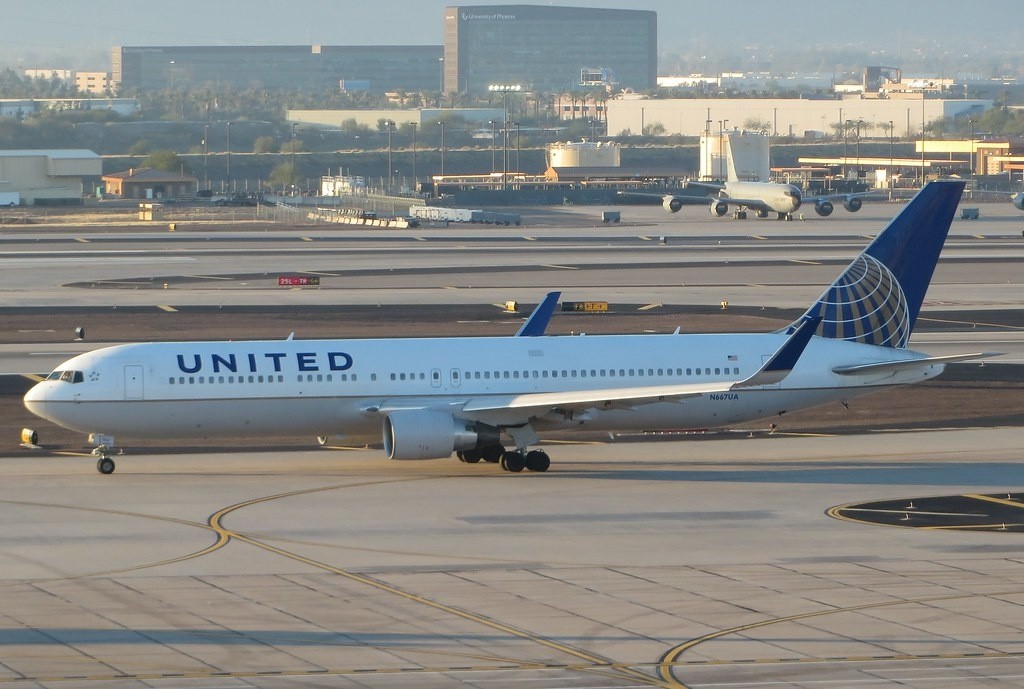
left=844, top=120, right=852, bottom=179
left=487, top=84, right=521, bottom=190
left=385, top=122, right=395, bottom=194
left=719, top=121, right=722, bottom=183
left=437, top=120, right=446, bottom=176
left=203, top=125, right=211, bottom=189
left=705, top=120, right=712, bottom=181
left=291, top=123, right=299, bottom=198
left=513, top=122, right=520, bottom=183
left=856, top=120, right=864, bottom=179
left=227, top=122, right=233, bottom=188
left=890, top=120, right=893, bottom=189
left=409, top=122, right=420, bottom=188
left=724, top=120, right=728, bottom=128
left=488, top=120, right=496, bottom=176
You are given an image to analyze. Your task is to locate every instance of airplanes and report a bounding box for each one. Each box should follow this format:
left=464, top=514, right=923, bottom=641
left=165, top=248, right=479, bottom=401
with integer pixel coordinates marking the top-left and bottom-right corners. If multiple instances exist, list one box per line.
left=22, top=181, right=1023, bottom=469
left=618, top=129, right=878, bottom=220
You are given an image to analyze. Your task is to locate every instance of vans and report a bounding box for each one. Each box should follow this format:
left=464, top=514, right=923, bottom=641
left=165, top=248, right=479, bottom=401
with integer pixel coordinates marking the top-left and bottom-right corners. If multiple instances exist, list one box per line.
left=195, top=190, right=211, bottom=196
left=0, top=192, right=20, bottom=207
